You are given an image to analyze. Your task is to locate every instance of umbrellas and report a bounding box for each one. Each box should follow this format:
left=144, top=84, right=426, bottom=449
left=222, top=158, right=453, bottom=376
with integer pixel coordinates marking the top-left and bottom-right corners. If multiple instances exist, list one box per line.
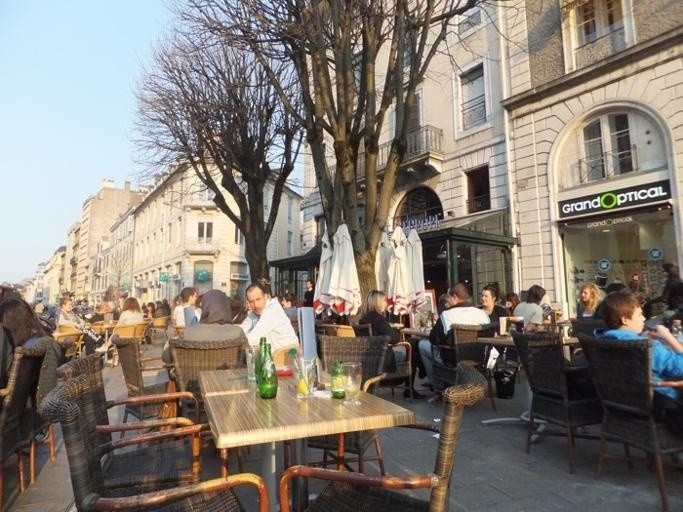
left=312, top=222, right=428, bottom=323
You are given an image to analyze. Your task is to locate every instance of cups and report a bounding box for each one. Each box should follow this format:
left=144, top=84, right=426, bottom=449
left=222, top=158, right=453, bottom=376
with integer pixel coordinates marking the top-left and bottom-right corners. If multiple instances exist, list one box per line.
left=294, top=395, right=318, bottom=420
left=245, top=346, right=257, bottom=379
left=294, top=358, right=316, bottom=396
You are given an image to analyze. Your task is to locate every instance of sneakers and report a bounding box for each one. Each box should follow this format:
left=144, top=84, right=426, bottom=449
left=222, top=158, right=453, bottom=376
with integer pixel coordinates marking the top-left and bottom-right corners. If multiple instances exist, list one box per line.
left=94, top=344, right=109, bottom=352
left=35, top=427, right=50, bottom=443
left=404, top=389, right=427, bottom=401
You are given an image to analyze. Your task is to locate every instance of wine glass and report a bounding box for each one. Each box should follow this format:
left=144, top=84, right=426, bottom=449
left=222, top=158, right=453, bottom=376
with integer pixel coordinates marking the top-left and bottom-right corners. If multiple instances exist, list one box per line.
left=343, top=362, right=363, bottom=408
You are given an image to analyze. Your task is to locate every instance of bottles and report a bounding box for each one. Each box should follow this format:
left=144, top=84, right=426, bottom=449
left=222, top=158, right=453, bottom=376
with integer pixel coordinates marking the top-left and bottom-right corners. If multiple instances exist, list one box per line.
left=330, top=358, right=343, bottom=399
left=670, top=319, right=682, bottom=337
left=258, top=400, right=279, bottom=430
left=255, top=336, right=267, bottom=384
left=330, top=398, right=348, bottom=423
left=257, top=344, right=277, bottom=399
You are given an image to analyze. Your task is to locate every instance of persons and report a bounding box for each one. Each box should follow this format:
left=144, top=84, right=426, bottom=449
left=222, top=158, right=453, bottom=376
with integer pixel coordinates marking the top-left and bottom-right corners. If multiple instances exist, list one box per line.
left=359, top=279, right=683, bottom=427
left=0, top=280, right=316, bottom=415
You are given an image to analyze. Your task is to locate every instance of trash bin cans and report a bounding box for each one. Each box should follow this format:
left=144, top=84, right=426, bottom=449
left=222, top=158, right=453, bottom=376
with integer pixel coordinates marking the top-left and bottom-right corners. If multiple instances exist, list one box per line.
left=494, top=371, right=515, bottom=400
